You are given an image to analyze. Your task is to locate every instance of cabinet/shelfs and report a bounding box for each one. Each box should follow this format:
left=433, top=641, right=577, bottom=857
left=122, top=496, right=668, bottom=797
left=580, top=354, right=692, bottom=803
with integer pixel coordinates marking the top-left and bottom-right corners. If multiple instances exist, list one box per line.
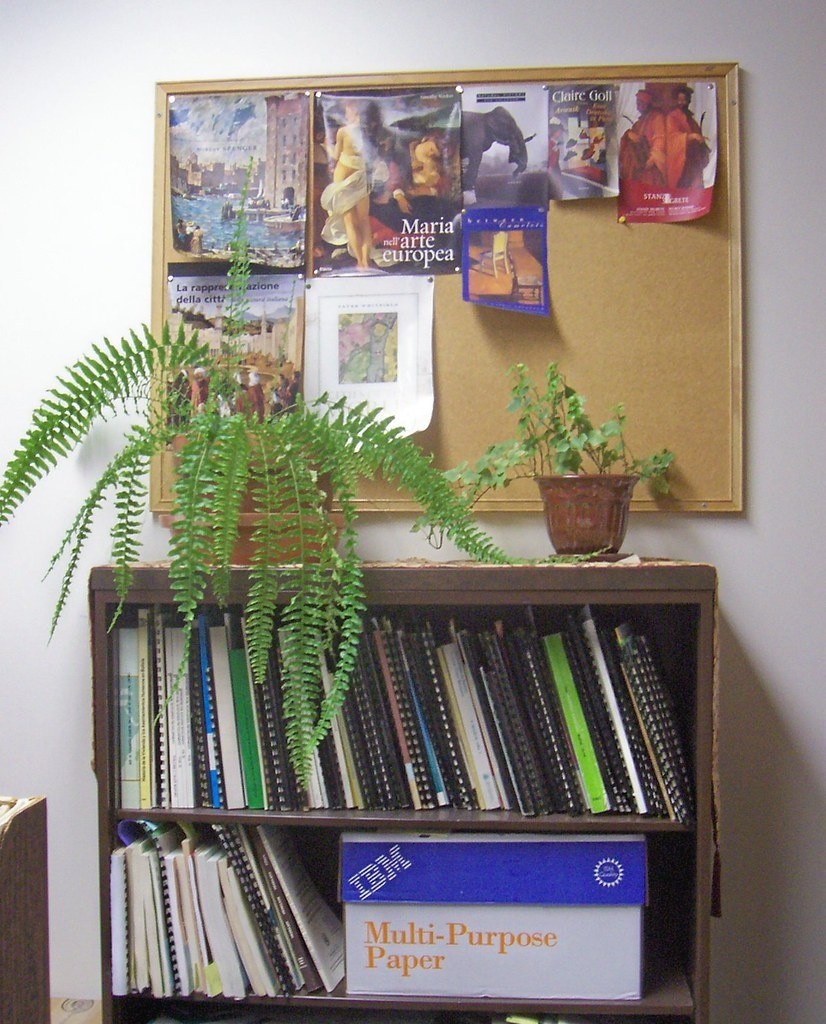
left=84, top=558, right=722, bottom=1024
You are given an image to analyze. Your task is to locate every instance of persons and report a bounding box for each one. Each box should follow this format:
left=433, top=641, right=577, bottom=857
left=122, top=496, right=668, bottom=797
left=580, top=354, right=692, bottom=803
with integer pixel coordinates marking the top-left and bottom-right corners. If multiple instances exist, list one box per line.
left=323, top=102, right=411, bottom=270
left=619, top=86, right=712, bottom=188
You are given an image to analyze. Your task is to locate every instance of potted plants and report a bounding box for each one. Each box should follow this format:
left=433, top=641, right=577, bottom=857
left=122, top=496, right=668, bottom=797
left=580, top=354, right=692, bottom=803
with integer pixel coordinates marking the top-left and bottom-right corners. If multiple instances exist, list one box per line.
left=458, top=361, right=673, bottom=551
left=0, top=156, right=612, bottom=790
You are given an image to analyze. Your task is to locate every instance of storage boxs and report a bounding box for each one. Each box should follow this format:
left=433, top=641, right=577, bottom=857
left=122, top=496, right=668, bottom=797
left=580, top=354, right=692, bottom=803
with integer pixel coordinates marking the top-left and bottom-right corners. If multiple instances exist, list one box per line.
left=335, top=831, right=648, bottom=1002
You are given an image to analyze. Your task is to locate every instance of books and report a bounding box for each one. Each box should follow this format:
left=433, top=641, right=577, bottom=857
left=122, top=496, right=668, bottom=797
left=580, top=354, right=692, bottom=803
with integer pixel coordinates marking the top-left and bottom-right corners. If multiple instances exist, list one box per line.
left=109, top=825, right=347, bottom=1000
left=118, top=601, right=696, bottom=822
